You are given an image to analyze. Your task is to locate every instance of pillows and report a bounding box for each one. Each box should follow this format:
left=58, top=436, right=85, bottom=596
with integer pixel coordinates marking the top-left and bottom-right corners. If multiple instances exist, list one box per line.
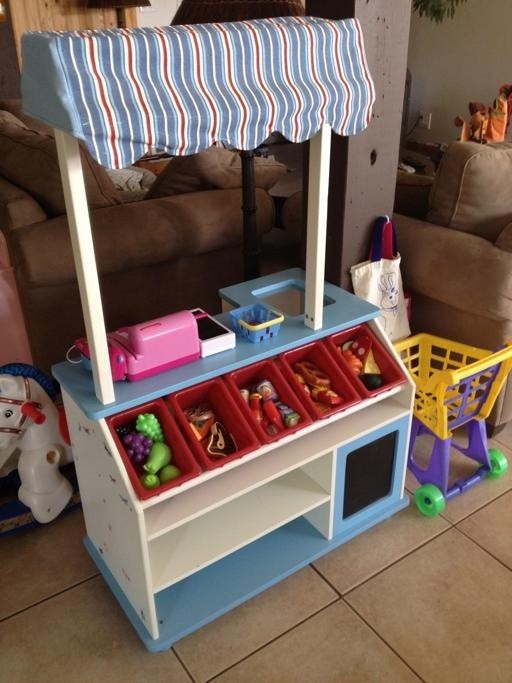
left=142, top=150, right=288, bottom=200
left=427, top=140, right=511, bottom=244
left=0, top=108, right=123, bottom=209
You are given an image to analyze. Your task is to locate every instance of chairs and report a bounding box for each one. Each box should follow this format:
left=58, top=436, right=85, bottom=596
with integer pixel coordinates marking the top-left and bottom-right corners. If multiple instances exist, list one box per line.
left=389, top=135, right=511, bottom=437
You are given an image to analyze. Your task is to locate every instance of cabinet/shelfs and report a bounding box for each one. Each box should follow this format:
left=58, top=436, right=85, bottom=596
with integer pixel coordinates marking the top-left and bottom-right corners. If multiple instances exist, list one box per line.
left=51, top=268, right=417, bottom=657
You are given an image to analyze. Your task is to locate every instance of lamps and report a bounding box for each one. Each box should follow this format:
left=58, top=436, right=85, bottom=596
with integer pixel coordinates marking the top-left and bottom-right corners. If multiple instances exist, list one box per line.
left=81, top=0, right=152, bottom=28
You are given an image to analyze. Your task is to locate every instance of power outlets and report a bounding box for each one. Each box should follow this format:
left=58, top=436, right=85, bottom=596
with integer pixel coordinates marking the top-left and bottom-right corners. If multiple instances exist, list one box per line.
left=418, top=109, right=432, bottom=129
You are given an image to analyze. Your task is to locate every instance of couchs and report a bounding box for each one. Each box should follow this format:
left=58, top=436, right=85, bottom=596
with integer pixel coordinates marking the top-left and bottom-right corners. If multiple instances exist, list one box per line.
left=0, top=99, right=310, bottom=374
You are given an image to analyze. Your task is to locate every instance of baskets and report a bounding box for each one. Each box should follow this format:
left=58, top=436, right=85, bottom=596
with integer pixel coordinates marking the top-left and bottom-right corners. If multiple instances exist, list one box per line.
left=229, top=303, right=285, bottom=344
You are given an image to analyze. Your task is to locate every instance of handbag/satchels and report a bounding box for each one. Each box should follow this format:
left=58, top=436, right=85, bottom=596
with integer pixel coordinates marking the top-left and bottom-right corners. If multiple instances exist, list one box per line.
left=350, top=215, right=412, bottom=342
